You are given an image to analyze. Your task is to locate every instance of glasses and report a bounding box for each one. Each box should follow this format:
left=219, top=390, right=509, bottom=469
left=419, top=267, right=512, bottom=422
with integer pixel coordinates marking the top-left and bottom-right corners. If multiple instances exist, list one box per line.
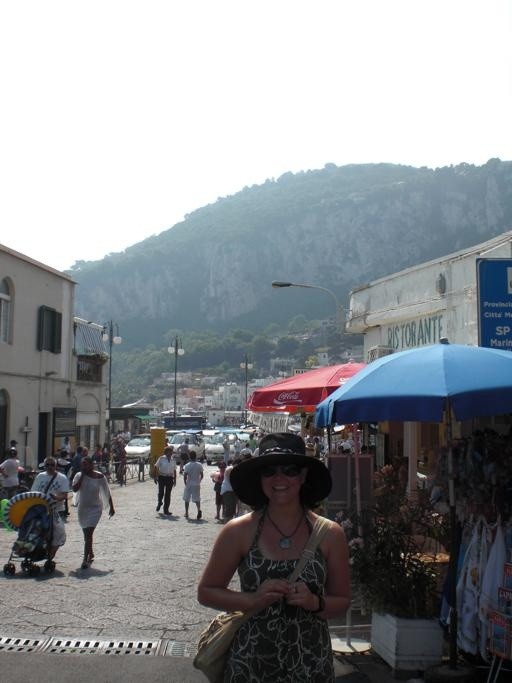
left=256, top=465, right=303, bottom=476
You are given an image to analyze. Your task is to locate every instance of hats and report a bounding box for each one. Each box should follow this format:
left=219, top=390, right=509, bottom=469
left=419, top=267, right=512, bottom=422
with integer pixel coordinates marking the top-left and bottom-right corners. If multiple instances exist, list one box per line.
left=229, top=433, right=332, bottom=507
left=164, top=447, right=173, bottom=452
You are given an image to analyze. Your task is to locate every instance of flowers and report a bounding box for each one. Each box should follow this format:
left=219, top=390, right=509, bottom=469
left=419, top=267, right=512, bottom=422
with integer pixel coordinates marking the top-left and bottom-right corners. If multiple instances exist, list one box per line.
left=333, top=463, right=458, bottom=618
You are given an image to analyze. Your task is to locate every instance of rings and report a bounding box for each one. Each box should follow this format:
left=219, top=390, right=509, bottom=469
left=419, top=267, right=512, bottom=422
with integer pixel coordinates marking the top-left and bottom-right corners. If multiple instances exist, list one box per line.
left=294, top=587, right=298, bottom=593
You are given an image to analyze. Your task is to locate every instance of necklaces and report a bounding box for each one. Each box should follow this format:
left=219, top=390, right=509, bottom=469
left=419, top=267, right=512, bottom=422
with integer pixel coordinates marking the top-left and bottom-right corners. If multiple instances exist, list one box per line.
left=267, top=511, right=306, bottom=549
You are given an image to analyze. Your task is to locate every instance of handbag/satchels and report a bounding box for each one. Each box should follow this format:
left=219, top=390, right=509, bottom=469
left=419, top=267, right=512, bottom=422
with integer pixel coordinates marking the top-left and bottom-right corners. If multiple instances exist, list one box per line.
left=193, top=610, right=245, bottom=683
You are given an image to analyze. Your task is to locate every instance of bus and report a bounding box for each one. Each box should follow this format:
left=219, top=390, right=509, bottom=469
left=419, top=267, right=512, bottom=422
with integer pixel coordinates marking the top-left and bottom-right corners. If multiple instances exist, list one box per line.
left=164, top=416, right=207, bottom=429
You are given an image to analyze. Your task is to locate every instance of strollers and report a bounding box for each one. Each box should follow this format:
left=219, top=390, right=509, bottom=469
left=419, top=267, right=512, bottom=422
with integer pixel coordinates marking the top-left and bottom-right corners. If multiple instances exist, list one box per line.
left=2, top=502, right=59, bottom=578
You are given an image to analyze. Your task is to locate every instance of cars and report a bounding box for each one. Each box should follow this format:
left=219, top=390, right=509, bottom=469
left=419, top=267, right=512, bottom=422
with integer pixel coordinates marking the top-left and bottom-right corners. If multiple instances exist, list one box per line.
left=124, top=424, right=259, bottom=465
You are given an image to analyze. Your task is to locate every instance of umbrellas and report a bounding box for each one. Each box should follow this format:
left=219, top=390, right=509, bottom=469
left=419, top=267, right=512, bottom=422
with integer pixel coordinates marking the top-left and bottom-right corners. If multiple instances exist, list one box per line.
left=243, top=357, right=369, bottom=457
left=315, top=337, right=512, bottom=667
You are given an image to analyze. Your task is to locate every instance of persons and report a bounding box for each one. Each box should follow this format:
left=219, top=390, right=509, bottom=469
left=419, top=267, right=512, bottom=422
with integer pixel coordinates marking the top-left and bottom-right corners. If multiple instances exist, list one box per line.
left=0, top=429, right=132, bottom=506
left=72, top=455, right=115, bottom=568
left=153, top=424, right=427, bottom=522
left=197, top=432, right=350, bottom=683
left=14, top=527, right=41, bottom=556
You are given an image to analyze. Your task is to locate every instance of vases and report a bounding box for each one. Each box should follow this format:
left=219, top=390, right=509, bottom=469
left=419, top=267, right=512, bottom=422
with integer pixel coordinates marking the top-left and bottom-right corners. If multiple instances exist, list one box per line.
left=371, top=608, right=444, bottom=677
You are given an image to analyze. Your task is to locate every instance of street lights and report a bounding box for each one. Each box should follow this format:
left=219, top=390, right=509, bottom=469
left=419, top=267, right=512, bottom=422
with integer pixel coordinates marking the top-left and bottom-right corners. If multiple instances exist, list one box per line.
left=240, top=352, right=253, bottom=427
left=168, top=333, right=185, bottom=429
left=101, top=318, right=121, bottom=453
left=279, top=366, right=288, bottom=381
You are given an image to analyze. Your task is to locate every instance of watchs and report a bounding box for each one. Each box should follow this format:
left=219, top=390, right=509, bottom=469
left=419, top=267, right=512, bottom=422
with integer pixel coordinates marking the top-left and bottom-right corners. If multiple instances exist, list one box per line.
left=310, top=594, right=325, bottom=614
